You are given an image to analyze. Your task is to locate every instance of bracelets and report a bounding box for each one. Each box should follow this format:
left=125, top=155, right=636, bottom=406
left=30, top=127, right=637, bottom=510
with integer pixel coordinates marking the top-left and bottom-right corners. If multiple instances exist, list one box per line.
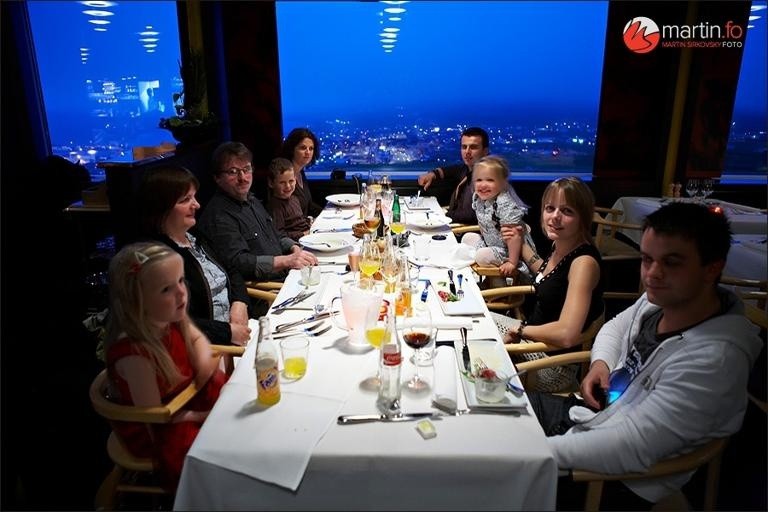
left=433, top=169, right=440, bottom=180
left=526, top=254, right=540, bottom=267
left=516, top=319, right=528, bottom=338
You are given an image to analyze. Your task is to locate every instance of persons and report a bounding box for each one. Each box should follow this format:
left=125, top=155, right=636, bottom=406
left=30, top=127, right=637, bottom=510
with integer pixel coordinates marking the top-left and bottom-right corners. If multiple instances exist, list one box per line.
left=417, top=126, right=490, bottom=245
left=82, top=239, right=233, bottom=474
left=264, top=157, right=312, bottom=238
left=483, top=177, right=604, bottom=393
left=191, top=140, right=320, bottom=286
left=458, top=154, right=538, bottom=282
left=124, top=162, right=261, bottom=348
left=527, top=198, right=763, bottom=510
left=281, top=127, right=324, bottom=221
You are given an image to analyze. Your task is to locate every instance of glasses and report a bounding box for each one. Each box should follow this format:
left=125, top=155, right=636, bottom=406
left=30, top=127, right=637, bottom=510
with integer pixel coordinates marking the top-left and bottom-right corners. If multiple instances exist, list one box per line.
left=219, top=165, right=254, bottom=176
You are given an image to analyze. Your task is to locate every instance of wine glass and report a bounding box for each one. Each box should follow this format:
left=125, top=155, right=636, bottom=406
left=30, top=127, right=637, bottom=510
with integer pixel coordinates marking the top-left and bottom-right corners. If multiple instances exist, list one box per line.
left=402, top=307, right=433, bottom=390
left=359, top=190, right=407, bottom=378
left=348, top=255, right=359, bottom=282
left=680, top=177, right=717, bottom=204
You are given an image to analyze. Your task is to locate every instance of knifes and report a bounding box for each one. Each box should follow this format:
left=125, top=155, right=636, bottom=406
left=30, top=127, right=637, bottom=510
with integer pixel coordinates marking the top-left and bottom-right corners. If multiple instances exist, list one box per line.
left=337, top=411, right=443, bottom=424
left=459, top=327, right=472, bottom=373
left=275, top=291, right=317, bottom=315
left=447, top=270, right=456, bottom=295
left=421, top=280, right=430, bottom=303
left=276, top=310, right=340, bottom=330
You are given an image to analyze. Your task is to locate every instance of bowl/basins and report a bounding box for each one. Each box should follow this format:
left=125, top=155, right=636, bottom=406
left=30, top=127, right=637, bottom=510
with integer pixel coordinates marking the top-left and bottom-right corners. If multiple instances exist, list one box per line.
left=475, top=369, right=506, bottom=404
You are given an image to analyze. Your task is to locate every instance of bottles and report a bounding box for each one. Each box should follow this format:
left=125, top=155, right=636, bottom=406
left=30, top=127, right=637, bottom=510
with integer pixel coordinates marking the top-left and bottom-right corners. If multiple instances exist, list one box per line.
left=376, top=314, right=401, bottom=408
left=391, top=195, right=401, bottom=222
left=359, top=182, right=369, bottom=218
left=373, top=199, right=384, bottom=237
left=394, top=256, right=413, bottom=316
left=382, top=174, right=389, bottom=191
left=255, top=316, right=280, bottom=403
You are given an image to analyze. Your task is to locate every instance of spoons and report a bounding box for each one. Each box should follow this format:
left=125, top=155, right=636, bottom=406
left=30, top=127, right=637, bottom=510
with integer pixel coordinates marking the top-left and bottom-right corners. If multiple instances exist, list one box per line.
left=290, top=239, right=332, bottom=247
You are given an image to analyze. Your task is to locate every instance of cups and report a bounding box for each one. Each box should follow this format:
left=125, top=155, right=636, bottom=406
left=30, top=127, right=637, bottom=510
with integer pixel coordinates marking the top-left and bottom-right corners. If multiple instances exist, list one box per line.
left=412, top=238, right=431, bottom=258
left=410, top=322, right=438, bottom=364
left=301, top=266, right=321, bottom=286
left=279, top=338, right=310, bottom=378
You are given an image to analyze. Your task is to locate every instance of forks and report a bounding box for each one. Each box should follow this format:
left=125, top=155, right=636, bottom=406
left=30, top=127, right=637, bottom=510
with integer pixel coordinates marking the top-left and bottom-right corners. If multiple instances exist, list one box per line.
left=425, top=212, right=432, bottom=225
left=273, top=325, right=332, bottom=341
left=457, top=273, right=465, bottom=299
left=273, top=290, right=305, bottom=308
left=321, top=270, right=350, bottom=275
left=266, top=321, right=326, bottom=334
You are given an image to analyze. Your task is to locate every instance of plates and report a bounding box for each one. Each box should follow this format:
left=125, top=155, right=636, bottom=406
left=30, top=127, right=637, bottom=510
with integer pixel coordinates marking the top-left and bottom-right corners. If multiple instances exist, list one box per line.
left=406, top=213, right=452, bottom=229
left=298, top=233, right=357, bottom=252
left=453, top=339, right=527, bottom=410
left=430, top=277, right=485, bottom=317
left=324, top=212, right=354, bottom=219
left=406, top=197, right=430, bottom=210
left=326, top=193, right=360, bottom=206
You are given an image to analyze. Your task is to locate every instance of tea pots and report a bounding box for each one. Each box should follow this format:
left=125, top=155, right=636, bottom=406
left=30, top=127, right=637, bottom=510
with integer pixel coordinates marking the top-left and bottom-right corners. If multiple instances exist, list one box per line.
left=330, top=281, right=383, bottom=345
